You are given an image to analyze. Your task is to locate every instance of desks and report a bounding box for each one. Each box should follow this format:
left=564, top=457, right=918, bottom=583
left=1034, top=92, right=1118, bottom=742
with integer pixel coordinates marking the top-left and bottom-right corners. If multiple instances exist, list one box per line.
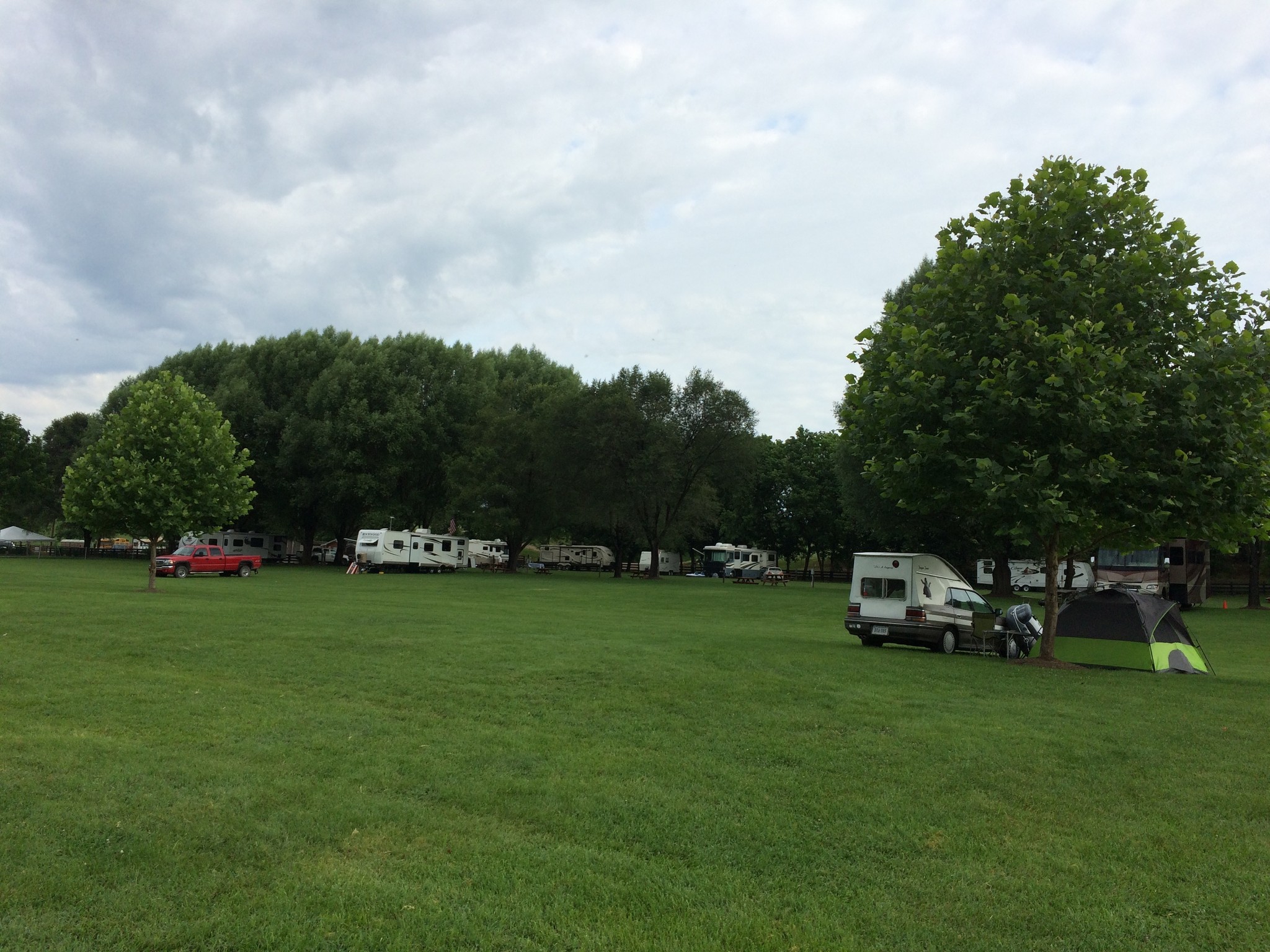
left=736, top=577, right=755, bottom=584
left=632, top=571, right=647, bottom=578
left=762, top=574, right=791, bottom=586
left=492, top=564, right=504, bottom=573
left=537, top=568, right=552, bottom=574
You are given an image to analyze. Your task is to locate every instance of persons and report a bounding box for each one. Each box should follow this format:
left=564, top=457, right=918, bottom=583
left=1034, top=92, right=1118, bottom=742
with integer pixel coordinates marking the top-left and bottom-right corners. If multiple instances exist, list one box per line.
left=322, top=551, right=326, bottom=563
left=488, top=555, right=505, bottom=562
left=525, top=554, right=529, bottom=570
left=11, top=541, right=31, bottom=556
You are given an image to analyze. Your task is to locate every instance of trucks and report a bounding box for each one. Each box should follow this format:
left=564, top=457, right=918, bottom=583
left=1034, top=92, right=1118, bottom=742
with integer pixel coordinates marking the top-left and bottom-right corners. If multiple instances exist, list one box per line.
left=693, top=542, right=777, bottom=579
left=1091, top=538, right=1212, bottom=610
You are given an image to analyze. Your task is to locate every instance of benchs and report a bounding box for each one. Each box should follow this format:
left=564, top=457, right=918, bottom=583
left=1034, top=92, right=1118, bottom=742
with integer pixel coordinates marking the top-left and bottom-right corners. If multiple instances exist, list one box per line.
left=732, top=579, right=790, bottom=586
left=534, top=572, right=552, bottom=575
left=491, top=568, right=510, bottom=572
left=630, top=575, right=644, bottom=579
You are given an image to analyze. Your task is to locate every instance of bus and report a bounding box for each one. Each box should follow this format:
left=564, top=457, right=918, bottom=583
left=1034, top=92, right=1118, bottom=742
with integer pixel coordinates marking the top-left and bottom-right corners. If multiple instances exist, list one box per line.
left=132, top=539, right=166, bottom=554
left=96, top=538, right=130, bottom=551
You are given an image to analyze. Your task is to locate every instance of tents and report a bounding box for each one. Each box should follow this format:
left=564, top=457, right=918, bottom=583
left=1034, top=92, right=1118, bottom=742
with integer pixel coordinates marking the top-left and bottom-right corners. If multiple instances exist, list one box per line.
left=0, top=526, right=56, bottom=558
left=1027, top=583, right=1217, bottom=675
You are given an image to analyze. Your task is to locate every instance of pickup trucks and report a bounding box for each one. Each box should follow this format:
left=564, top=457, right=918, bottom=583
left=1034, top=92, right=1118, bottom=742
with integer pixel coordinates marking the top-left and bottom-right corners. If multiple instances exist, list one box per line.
left=295, top=547, right=350, bottom=566
left=844, top=552, right=1021, bottom=659
left=148, top=545, right=262, bottom=579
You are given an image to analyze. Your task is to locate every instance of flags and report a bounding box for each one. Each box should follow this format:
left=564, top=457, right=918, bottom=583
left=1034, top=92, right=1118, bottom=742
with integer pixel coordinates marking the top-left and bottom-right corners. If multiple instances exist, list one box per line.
left=450, top=518, right=457, bottom=534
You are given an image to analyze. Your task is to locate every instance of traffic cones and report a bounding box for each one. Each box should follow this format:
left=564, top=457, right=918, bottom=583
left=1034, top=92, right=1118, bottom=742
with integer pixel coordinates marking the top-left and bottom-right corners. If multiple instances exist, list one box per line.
left=1223, top=599, right=1228, bottom=609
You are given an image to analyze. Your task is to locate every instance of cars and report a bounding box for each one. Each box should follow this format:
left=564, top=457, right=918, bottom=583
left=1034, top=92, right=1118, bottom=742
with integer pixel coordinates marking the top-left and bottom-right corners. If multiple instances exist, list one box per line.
left=0, top=541, right=16, bottom=549
left=763, top=567, right=784, bottom=580
left=112, top=544, right=125, bottom=551
